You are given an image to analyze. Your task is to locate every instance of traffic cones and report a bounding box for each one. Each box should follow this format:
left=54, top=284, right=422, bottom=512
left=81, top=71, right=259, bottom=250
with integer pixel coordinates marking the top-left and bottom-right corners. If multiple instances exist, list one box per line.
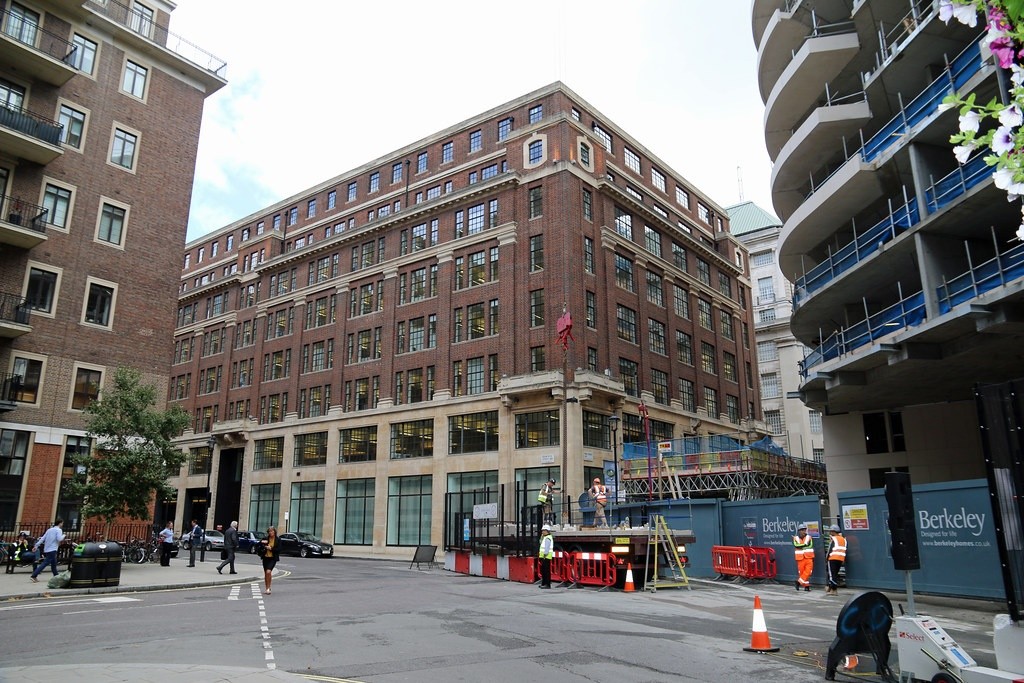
left=743, top=596, right=780, bottom=652
left=621, top=563, right=638, bottom=592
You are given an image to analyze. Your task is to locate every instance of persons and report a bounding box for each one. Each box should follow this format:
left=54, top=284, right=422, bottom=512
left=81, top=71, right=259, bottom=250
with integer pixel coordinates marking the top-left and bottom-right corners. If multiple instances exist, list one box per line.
left=30, top=518, right=67, bottom=583
left=539, top=525, right=553, bottom=589
left=824, top=524, right=848, bottom=596
left=216, top=521, right=239, bottom=574
left=588, top=478, right=611, bottom=527
left=186, top=520, right=202, bottom=567
left=159, top=521, right=174, bottom=566
left=793, top=524, right=815, bottom=591
left=257, top=526, right=281, bottom=595
left=11, top=532, right=41, bottom=574
left=537, top=478, right=556, bottom=525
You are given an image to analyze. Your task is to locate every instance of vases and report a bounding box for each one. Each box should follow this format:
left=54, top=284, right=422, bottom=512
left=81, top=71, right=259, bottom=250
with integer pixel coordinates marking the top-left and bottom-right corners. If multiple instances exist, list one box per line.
left=9, top=214, right=22, bottom=224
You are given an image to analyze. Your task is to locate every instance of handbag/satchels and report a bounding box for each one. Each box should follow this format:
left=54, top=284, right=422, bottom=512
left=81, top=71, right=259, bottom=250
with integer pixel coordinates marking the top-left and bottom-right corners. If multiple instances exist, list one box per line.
left=254, top=541, right=267, bottom=559
left=221, top=551, right=228, bottom=560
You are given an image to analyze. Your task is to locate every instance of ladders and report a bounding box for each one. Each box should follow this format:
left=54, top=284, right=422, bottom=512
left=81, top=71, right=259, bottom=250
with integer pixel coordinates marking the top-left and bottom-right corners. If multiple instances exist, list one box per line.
left=643, top=512, right=692, bottom=593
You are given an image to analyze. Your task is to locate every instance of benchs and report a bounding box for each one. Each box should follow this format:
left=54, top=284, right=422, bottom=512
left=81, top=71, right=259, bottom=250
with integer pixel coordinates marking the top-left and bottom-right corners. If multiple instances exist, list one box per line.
left=5, top=543, right=73, bottom=573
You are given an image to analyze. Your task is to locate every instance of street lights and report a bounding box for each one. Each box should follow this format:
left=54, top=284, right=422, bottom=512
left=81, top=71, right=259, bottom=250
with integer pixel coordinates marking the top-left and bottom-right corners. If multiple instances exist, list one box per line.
left=199, top=437, right=220, bottom=560
left=609, top=416, right=622, bottom=528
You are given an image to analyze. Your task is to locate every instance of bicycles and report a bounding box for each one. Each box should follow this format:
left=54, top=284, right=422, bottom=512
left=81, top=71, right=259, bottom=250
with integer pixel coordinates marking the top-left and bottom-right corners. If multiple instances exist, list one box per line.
left=0, top=531, right=160, bottom=568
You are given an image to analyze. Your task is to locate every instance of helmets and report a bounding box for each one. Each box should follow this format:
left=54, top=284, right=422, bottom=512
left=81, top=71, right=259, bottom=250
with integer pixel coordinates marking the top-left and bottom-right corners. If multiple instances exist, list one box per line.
left=594, top=478, right=600, bottom=483
left=541, top=525, right=551, bottom=530
left=549, top=477, right=556, bottom=485
left=18, top=532, right=26, bottom=537
left=828, top=524, right=841, bottom=532
left=798, top=524, right=807, bottom=530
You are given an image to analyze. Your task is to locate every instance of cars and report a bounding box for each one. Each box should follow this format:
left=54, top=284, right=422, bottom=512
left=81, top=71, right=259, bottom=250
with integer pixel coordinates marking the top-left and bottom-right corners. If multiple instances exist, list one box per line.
left=181, top=529, right=225, bottom=551
left=234, top=531, right=267, bottom=554
left=278, top=531, right=334, bottom=558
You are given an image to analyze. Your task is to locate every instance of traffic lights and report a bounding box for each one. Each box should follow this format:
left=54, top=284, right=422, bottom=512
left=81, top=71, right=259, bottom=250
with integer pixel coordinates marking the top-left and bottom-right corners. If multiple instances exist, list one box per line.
left=884, top=471, right=921, bottom=570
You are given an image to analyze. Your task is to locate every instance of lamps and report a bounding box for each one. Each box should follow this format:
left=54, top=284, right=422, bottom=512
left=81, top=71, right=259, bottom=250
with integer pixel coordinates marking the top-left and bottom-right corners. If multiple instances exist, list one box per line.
left=571, top=159, right=576, bottom=164
left=552, top=159, right=557, bottom=164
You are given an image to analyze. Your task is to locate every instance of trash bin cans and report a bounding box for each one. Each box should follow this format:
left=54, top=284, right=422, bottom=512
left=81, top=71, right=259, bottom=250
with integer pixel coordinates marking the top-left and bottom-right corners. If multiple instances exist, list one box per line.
left=69, top=541, right=100, bottom=587
left=107, top=541, right=122, bottom=586
left=94, top=541, right=109, bottom=587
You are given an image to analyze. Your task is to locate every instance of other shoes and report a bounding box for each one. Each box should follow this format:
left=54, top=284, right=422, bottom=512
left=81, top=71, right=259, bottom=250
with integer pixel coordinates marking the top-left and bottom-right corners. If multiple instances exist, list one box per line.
left=539, top=584, right=551, bottom=588
left=795, top=582, right=800, bottom=590
left=230, top=571, right=237, bottom=574
left=30, top=576, right=36, bottom=582
left=805, top=586, right=810, bottom=591
left=216, top=566, right=222, bottom=574
left=265, top=588, right=272, bottom=595
left=825, top=585, right=837, bottom=595
left=187, top=565, right=195, bottom=567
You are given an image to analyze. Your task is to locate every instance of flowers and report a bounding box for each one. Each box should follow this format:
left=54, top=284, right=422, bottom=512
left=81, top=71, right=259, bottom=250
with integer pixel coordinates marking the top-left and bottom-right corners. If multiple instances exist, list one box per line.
left=9, top=197, right=22, bottom=215
